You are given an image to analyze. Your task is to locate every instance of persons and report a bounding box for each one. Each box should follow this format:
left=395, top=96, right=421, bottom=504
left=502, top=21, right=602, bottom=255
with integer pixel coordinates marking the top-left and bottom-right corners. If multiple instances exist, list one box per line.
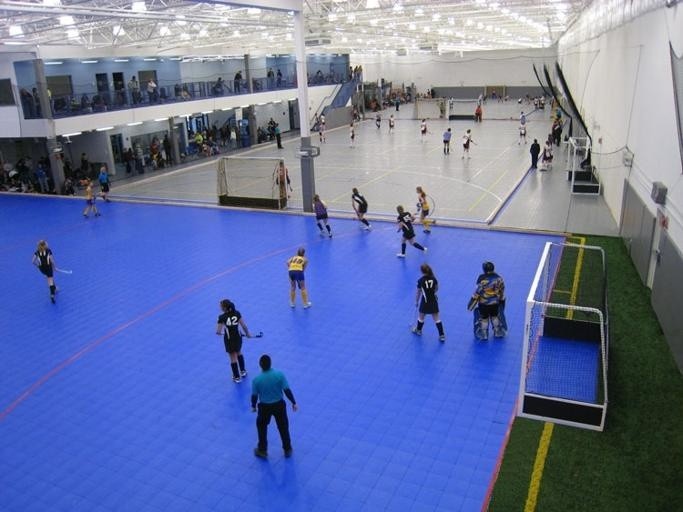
left=549, top=99, right=562, bottom=146
left=350, top=103, right=395, bottom=142
left=351, top=187, right=372, bottom=231
left=395, top=205, right=427, bottom=257
left=466, top=260, right=509, bottom=341
left=415, top=186, right=436, bottom=233
left=460, top=129, right=473, bottom=159
left=443, top=128, right=452, bottom=154
left=216, top=298, right=252, bottom=383
left=213, top=70, right=242, bottom=94
left=519, top=92, right=546, bottom=110
left=420, top=120, right=426, bottom=135
left=124, top=126, right=238, bottom=174
left=2, top=152, right=113, bottom=218
left=492, top=88, right=497, bottom=96
left=307, top=66, right=362, bottom=85
left=277, top=161, right=292, bottom=197
left=312, top=195, right=333, bottom=238
left=21, top=75, right=158, bottom=118
left=258, top=117, right=284, bottom=149
left=439, top=95, right=453, bottom=117
left=474, top=95, right=488, bottom=122
left=369, top=89, right=431, bottom=112
left=411, top=263, right=446, bottom=342
left=267, top=68, right=282, bottom=85
left=530, top=134, right=553, bottom=171
left=174, top=82, right=191, bottom=100
left=32, top=239, right=59, bottom=304
left=286, top=247, right=312, bottom=309
left=315, top=112, right=326, bottom=143
left=518, top=112, right=527, bottom=145
left=250, top=355, right=297, bottom=459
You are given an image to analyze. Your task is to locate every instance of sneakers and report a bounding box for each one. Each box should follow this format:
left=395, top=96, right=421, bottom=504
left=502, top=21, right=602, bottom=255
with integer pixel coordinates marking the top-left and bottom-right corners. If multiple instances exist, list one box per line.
left=396, top=219, right=436, bottom=258
left=285, top=447, right=292, bottom=457
left=50, top=295, right=56, bottom=304
left=254, top=448, right=268, bottom=457
left=440, top=334, right=444, bottom=341
left=232, top=375, right=242, bottom=383
left=240, top=369, right=247, bottom=376
left=317, top=223, right=372, bottom=240
left=304, top=302, right=311, bottom=308
left=481, top=328, right=505, bottom=340
left=412, top=327, right=422, bottom=336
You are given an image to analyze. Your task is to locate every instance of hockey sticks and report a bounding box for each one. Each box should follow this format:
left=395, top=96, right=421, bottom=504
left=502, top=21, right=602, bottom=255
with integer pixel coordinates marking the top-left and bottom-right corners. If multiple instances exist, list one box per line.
left=214, top=331, right=263, bottom=338
left=54, top=267, right=72, bottom=277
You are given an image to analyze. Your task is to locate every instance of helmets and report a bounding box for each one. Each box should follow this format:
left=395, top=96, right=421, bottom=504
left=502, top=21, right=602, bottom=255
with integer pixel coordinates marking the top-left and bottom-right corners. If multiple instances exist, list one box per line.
left=482, top=262, right=494, bottom=274
